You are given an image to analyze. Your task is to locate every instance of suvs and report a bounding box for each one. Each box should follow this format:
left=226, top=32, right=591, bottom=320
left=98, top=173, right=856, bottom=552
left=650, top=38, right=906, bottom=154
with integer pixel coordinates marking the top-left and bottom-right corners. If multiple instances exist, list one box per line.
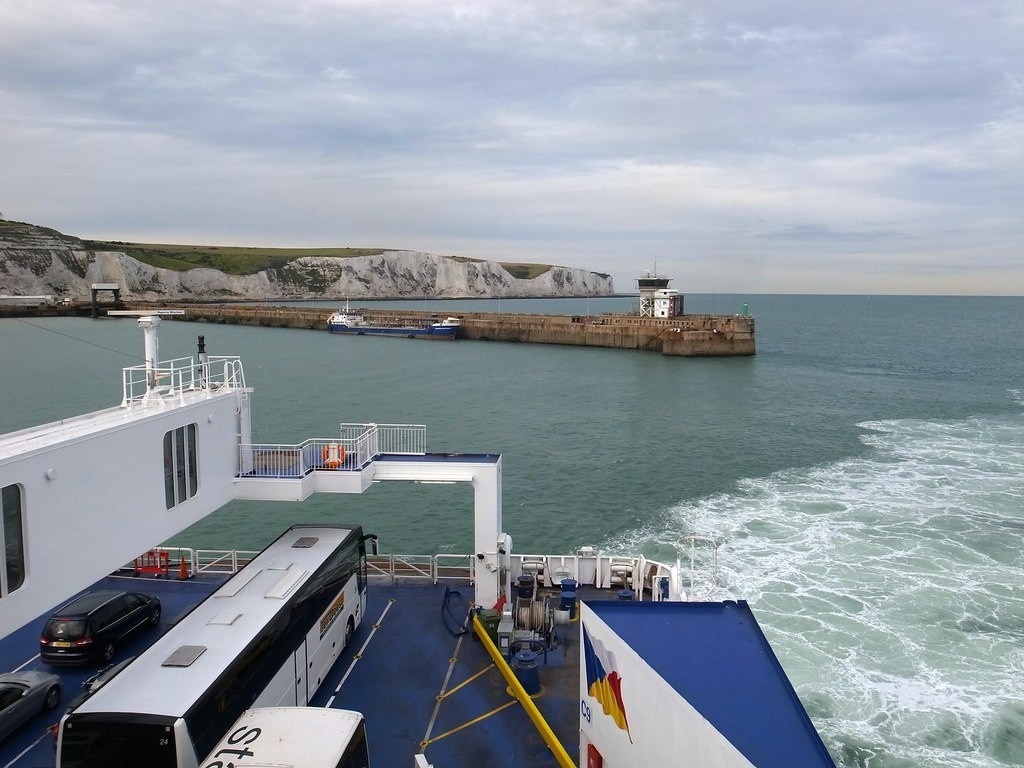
left=40, top=590, right=162, bottom=669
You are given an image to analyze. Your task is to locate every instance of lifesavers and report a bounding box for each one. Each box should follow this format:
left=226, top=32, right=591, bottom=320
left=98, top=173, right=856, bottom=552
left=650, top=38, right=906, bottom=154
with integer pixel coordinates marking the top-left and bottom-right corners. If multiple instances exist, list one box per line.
left=322, top=443, right=345, bottom=467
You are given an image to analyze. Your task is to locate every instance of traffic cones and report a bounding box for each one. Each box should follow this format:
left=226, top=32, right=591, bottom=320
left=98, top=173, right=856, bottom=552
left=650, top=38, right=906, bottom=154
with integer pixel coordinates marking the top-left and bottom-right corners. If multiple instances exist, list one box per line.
left=175, top=555, right=190, bottom=580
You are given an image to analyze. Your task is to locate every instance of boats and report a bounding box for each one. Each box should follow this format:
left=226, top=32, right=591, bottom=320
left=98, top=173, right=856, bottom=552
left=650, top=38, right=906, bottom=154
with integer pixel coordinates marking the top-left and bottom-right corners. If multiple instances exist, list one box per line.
left=325, top=295, right=461, bottom=340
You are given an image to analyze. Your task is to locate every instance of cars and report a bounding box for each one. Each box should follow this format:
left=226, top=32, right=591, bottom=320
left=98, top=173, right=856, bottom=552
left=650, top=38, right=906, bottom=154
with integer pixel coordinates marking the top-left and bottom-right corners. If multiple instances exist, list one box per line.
left=0, top=670, right=64, bottom=744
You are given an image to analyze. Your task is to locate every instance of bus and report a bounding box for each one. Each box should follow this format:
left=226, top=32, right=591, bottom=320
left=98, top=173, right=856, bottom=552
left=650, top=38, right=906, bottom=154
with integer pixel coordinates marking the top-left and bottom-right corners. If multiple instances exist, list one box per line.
left=55, top=523, right=379, bottom=768
left=197, top=705, right=371, bottom=768
